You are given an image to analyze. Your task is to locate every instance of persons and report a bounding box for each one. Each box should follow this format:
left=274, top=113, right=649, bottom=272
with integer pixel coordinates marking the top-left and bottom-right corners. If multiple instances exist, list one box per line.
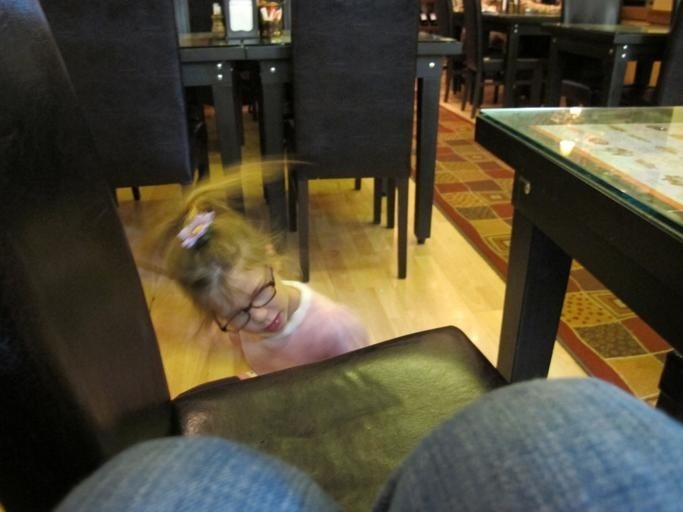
left=131, top=161, right=370, bottom=376
left=51, top=376, right=683, bottom=512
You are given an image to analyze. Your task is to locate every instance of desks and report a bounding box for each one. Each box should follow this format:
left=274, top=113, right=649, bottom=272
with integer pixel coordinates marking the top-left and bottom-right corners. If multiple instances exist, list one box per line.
left=474, top=103, right=682, bottom=423
left=538, top=21, right=672, bottom=109
left=178, top=32, right=463, bottom=245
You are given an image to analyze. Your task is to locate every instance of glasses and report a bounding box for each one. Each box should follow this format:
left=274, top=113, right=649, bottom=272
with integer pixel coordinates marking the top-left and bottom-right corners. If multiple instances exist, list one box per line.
left=212, top=268, right=276, bottom=333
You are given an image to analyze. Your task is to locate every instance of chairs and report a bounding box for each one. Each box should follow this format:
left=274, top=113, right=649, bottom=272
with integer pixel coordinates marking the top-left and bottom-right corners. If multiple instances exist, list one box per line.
left=287, top=2, right=418, bottom=283
left=435, top=1, right=560, bottom=111
left=41, top=0, right=209, bottom=206
left=0, top=0, right=512, bottom=512
